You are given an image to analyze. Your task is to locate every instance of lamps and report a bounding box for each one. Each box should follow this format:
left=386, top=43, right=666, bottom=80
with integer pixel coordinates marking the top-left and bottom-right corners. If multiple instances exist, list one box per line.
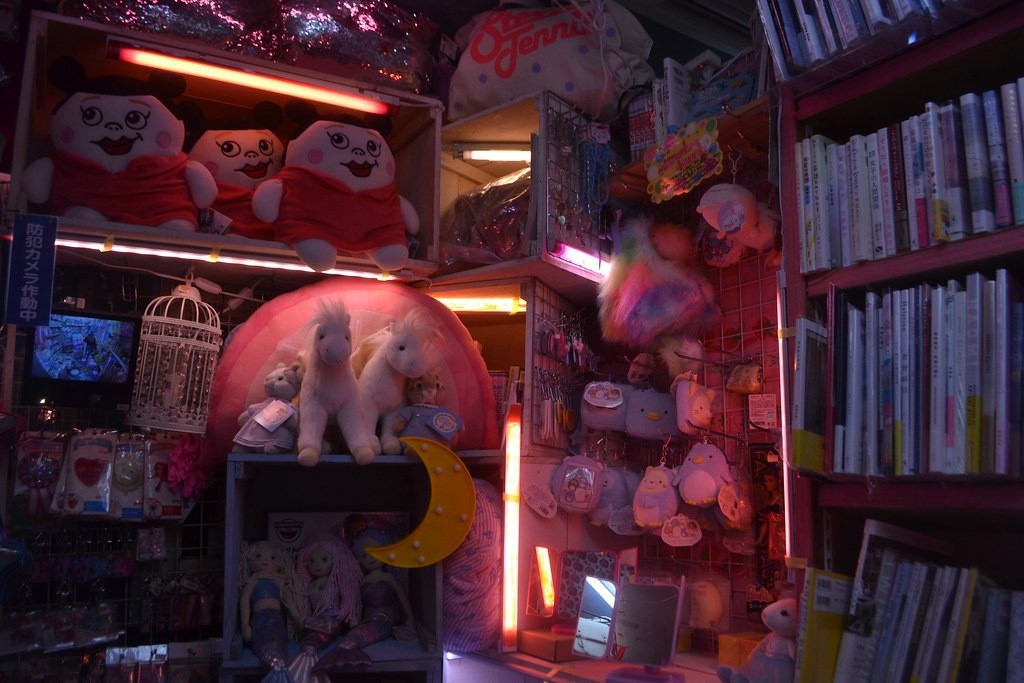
left=552, top=240, right=612, bottom=277
left=503, top=404, right=523, bottom=654
left=451, top=145, right=535, bottom=163
left=103, top=36, right=393, bottom=120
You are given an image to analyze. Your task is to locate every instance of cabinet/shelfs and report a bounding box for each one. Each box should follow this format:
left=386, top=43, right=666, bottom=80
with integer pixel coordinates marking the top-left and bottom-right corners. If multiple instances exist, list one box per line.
left=0, top=13, right=1024, bottom=683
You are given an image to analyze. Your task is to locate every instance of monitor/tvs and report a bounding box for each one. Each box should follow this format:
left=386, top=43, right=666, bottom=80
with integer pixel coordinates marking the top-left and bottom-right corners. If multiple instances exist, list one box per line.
left=21, top=309, right=141, bottom=408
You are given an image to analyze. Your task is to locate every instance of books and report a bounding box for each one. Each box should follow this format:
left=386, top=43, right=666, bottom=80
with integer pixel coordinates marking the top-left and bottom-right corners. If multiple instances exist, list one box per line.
left=755, top=0, right=1024, bottom=683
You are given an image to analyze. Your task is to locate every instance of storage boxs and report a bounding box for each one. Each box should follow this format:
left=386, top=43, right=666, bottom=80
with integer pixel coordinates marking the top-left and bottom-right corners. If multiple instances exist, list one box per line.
left=518, top=630, right=591, bottom=662
left=676, top=630, right=692, bottom=651
left=220, top=448, right=445, bottom=672
left=718, top=631, right=767, bottom=669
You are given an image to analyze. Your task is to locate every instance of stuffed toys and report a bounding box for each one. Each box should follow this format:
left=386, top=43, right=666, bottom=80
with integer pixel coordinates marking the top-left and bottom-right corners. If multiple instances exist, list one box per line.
left=311, top=529, right=415, bottom=675
left=292, top=537, right=363, bottom=683
left=1, top=1, right=797, bottom=683
left=239, top=538, right=299, bottom=683
